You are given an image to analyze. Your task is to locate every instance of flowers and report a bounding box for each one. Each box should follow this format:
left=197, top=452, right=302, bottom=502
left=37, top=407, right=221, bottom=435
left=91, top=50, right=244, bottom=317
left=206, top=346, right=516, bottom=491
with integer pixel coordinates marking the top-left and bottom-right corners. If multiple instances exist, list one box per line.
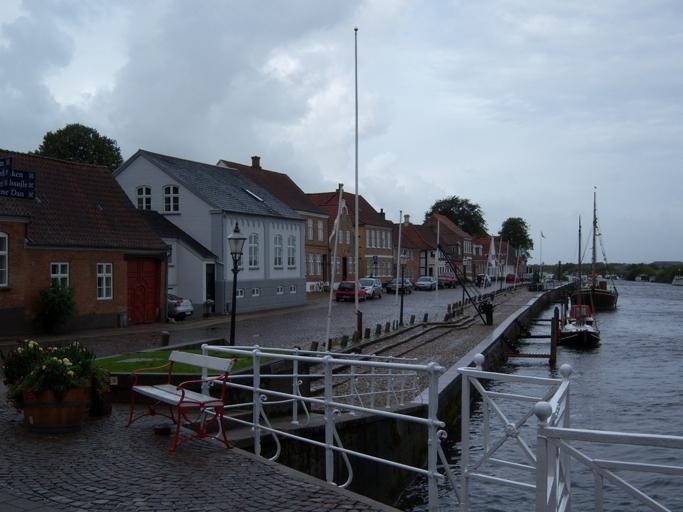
left=0, top=336, right=112, bottom=409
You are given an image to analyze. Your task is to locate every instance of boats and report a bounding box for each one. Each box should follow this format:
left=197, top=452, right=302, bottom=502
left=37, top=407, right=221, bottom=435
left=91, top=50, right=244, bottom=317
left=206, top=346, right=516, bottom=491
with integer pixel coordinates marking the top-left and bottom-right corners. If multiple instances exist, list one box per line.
left=635, top=272, right=655, bottom=282
left=671, top=275, right=682, bottom=285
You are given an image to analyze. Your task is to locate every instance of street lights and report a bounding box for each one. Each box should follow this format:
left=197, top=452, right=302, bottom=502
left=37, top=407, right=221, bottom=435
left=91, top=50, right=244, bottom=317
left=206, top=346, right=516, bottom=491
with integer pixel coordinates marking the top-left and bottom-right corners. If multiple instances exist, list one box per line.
left=228, top=218, right=247, bottom=345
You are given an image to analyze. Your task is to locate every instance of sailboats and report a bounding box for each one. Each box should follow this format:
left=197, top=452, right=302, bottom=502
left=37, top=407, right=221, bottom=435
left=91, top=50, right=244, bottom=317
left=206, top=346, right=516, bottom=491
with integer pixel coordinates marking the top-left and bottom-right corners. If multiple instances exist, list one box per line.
left=560, top=181, right=618, bottom=355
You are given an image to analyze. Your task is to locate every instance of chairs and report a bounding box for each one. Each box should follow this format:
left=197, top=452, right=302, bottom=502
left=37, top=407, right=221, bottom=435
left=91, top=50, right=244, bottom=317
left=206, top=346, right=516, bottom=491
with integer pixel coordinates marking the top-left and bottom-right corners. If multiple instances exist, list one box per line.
left=123, top=350, right=238, bottom=452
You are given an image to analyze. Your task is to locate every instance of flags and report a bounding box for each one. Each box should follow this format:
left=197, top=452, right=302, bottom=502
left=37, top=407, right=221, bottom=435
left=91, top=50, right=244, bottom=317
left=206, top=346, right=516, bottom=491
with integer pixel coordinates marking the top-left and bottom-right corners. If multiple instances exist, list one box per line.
left=327, top=198, right=354, bottom=257
left=488, top=237, right=495, bottom=268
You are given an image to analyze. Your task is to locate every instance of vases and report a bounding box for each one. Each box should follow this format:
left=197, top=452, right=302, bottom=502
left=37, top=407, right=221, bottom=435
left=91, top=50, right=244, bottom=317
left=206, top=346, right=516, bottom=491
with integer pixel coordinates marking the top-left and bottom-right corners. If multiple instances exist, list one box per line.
left=15, top=384, right=92, bottom=432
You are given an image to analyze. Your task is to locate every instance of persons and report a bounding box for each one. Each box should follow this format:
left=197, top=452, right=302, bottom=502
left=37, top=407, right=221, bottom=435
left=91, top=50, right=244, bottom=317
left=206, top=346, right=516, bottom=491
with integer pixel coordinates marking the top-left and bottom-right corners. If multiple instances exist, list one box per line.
left=484, top=294, right=493, bottom=325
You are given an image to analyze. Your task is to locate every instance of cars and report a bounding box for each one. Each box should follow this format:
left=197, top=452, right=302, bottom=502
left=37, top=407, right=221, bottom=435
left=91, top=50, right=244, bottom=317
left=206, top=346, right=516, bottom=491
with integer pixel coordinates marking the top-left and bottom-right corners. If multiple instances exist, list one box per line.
left=166, top=292, right=193, bottom=321
left=336, top=274, right=554, bottom=301
